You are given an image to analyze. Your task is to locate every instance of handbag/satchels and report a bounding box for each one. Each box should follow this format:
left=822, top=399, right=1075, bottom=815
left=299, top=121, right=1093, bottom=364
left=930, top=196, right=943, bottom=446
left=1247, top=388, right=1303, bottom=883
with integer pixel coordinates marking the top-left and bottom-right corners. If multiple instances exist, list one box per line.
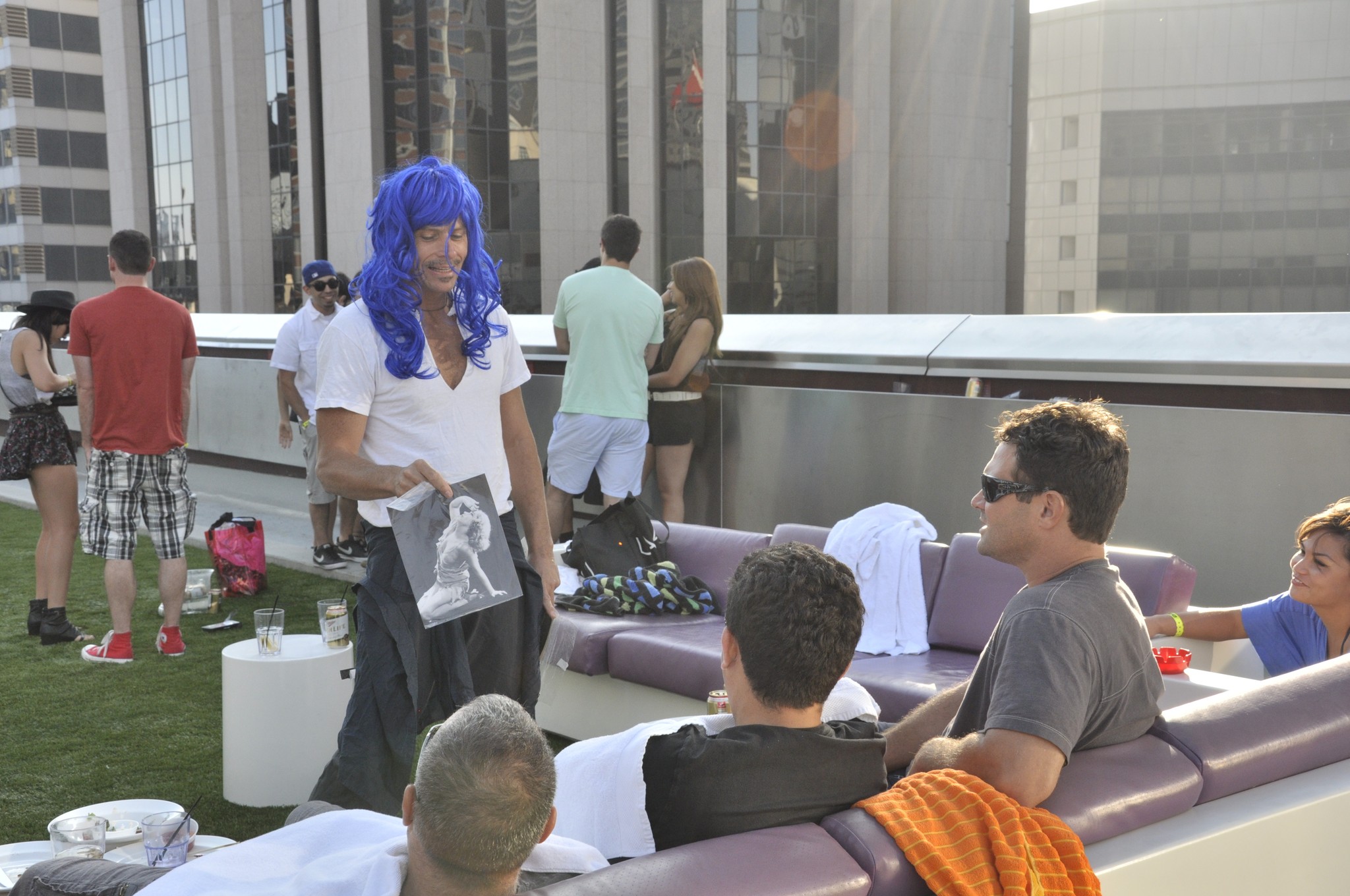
left=204, top=512, right=268, bottom=599
left=560, top=491, right=669, bottom=579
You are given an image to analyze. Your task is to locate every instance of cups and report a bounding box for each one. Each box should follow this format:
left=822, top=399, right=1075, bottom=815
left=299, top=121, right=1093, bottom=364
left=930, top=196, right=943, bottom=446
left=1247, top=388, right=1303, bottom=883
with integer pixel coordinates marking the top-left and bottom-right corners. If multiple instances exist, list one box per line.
left=254, top=608, right=284, bottom=657
left=317, top=598, right=349, bottom=649
left=141, top=811, right=190, bottom=868
left=50, top=816, right=106, bottom=859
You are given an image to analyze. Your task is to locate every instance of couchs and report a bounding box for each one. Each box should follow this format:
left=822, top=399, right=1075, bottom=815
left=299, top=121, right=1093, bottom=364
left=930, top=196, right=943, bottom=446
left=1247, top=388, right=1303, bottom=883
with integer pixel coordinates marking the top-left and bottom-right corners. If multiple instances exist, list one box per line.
left=515, top=518, right=1350, bottom=896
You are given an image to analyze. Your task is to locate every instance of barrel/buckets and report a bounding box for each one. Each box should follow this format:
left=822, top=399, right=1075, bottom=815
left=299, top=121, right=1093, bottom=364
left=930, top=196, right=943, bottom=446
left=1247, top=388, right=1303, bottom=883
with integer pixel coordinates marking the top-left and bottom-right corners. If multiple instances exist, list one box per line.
left=182, top=569, right=215, bottom=613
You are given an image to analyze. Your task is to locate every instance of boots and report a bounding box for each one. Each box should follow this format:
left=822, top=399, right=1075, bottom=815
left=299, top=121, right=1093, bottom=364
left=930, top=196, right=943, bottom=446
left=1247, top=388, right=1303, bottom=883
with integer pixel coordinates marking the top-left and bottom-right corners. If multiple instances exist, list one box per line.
left=26, top=598, right=47, bottom=636
left=40, top=606, right=85, bottom=645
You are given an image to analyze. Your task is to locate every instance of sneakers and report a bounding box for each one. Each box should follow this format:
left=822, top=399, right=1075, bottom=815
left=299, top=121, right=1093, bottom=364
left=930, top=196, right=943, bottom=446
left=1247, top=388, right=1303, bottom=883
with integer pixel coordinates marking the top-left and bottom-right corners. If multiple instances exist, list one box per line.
left=156, top=623, right=187, bottom=656
left=313, top=541, right=346, bottom=570
left=81, top=629, right=133, bottom=664
left=336, top=534, right=369, bottom=562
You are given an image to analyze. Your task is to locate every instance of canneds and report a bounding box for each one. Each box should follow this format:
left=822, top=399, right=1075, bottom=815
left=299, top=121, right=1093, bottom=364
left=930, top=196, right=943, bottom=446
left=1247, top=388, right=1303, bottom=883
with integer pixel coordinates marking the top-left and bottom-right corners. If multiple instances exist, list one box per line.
left=184, top=584, right=207, bottom=598
left=325, top=605, right=349, bottom=648
left=707, top=690, right=733, bottom=715
left=208, top=588, right=222, bottom=614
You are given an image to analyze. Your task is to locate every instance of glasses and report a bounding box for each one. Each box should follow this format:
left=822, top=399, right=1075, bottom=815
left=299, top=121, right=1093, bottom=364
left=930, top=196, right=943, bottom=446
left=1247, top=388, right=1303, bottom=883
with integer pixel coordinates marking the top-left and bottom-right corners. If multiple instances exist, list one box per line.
left=979, top=473, right=1073, bottom=503
left=308, top=278, right=339, bottom=292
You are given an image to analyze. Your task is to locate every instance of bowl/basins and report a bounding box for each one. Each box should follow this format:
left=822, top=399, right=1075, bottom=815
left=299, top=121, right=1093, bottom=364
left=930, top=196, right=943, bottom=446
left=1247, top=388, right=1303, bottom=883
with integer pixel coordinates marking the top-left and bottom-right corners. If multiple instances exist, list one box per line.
left=106, top=820, right=139, bottom=839
left=161, top=818, right=198, bottom=852
left=47, top=799, right=185, bottom=853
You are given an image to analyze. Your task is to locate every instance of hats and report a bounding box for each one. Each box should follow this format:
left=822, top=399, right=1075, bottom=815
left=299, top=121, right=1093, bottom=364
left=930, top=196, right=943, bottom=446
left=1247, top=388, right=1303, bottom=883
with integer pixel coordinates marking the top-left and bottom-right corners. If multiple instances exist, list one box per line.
left=302, top=260, right=339, bottom=286
left=17, top=291, right=75, bottom=311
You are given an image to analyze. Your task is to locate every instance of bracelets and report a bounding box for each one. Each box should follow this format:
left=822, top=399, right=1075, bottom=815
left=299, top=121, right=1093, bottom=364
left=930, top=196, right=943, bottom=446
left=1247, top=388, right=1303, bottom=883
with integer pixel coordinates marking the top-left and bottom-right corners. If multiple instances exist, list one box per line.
left=302, top=419, right=309, bottom=427
left=65, top=374, right=72, bottom=386
left=1167, top=612, right=1184, bottom=637
left=184, top=443, right=188, bottom=447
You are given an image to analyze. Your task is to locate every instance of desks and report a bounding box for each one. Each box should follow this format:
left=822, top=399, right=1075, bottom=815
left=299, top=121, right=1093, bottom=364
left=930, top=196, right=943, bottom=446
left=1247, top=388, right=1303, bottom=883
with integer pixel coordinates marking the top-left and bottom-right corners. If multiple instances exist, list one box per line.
left=221, top=635, right=355, bottom=808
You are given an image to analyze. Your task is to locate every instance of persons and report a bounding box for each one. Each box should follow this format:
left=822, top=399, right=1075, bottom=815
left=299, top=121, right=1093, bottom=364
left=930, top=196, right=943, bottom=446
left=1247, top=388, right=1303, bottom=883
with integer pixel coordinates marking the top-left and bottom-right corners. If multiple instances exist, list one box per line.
left=545, top=212, right=664, bottom=547
left=269, top=259, right=371, bottom=569
left=304, top=158, right=559, bottom=822
left=1143, top=494, right=1350, bottom=676
left=8, top=691, right=609, bottom=896
left=71, top=229, right=200, bottom=664
left=639, top=256, right=723, bottom=523
left=552, top=543, right=889, bottom=865
left=878, top=398, right=1165, bottom=807
left=0, top=289, right=95, bottom=645
left=417, top=496, right=507, bottom=622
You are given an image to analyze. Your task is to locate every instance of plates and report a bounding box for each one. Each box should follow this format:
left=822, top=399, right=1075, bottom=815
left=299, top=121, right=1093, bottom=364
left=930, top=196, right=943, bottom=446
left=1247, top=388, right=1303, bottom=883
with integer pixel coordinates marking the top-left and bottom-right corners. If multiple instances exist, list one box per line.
left=0, top=841, right=53, bottom=892
left=103, top=835, right=237, bottom=866
left=170, top=857, right=171, bottom=858
